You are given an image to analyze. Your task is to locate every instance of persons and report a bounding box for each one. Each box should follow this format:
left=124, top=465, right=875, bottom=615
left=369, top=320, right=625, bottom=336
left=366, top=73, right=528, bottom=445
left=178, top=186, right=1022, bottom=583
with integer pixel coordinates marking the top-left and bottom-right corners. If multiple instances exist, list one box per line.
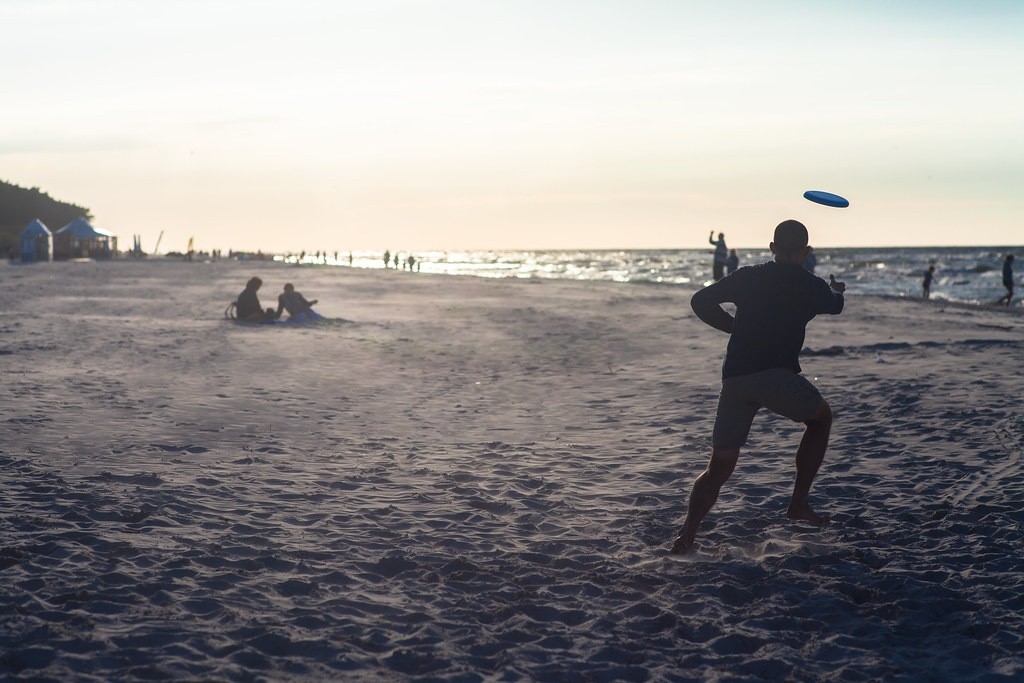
left=281, top=249, right=423, bottom=275
left=183, top=248, right=262, bottom=262
left=997, top=254, right=1014, bottom=305
left=236, top=277, right=276, bottom=321
left=275, top=284, right=332, bottom=326
left=922, top=266, right=938, bottom=299
left=673, top=221, right=845, bottom=552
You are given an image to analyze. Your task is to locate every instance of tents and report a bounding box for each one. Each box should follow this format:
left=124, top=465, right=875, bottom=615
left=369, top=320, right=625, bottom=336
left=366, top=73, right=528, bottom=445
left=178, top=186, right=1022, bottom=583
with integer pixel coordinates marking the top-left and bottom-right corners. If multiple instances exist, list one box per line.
left=18, top=216, right=117, bottom=262
